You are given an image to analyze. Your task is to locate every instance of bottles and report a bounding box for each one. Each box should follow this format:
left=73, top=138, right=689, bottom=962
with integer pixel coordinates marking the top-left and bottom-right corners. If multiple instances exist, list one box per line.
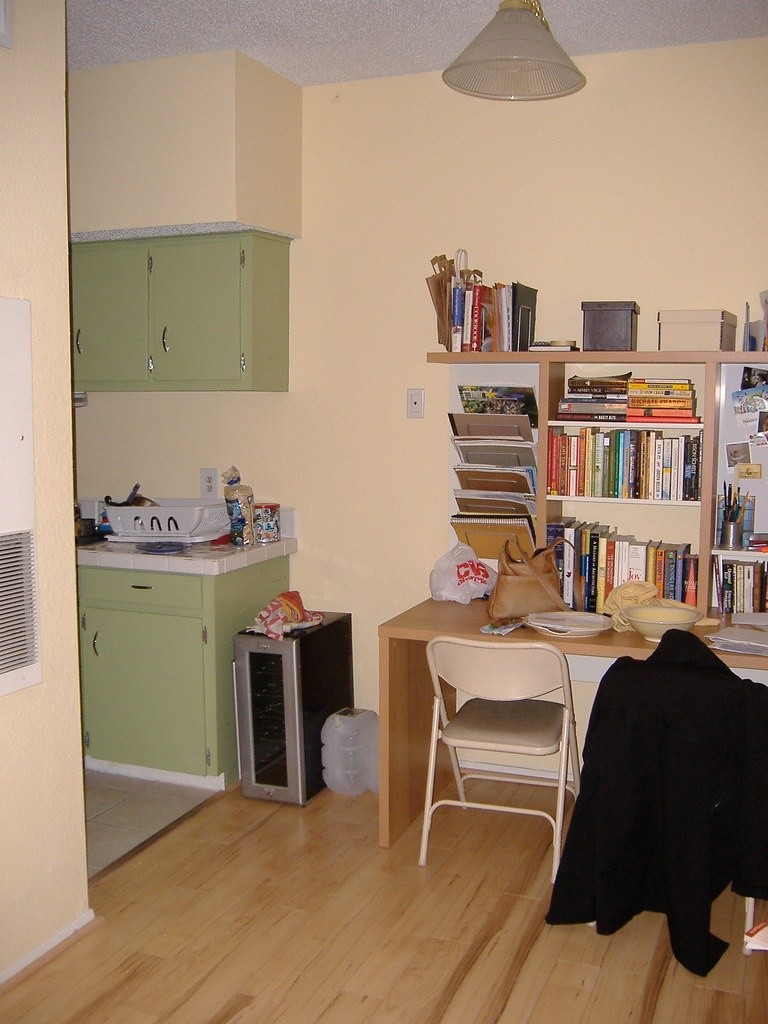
left=255, top=503, right=281, bottom=543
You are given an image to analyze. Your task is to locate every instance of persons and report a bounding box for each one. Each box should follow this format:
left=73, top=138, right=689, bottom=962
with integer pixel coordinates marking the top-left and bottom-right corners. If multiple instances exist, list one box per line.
left=742, top=370, right=750, bottom=389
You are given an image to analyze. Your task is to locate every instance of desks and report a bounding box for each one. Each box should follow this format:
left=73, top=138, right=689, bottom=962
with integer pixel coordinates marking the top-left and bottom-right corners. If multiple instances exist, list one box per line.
left=376, top=593, right=768, bottom=848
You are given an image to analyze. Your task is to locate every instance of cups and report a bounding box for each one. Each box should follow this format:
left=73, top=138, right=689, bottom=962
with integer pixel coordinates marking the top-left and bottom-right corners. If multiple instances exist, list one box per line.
left=719, top=521, right=742, bottom=550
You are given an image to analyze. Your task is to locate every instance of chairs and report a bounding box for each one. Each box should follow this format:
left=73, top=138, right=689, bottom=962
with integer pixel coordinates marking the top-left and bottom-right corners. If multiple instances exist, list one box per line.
left=417, top=634, right=581, bottom=884
left=542, top=629, right=768, bottom=978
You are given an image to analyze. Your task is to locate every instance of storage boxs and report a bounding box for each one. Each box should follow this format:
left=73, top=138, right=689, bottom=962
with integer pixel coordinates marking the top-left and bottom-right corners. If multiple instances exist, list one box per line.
left=581, top=301, right=640, bottom=350
left=657, top=308, right=738, bottom=351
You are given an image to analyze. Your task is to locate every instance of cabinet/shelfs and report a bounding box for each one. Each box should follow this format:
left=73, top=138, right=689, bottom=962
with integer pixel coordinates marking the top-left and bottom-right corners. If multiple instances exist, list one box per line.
left=68, top=228, right=294, bottom=393
left=427, top=351, right=768, bottom=617
left=76, top=555, right=290, bottom=790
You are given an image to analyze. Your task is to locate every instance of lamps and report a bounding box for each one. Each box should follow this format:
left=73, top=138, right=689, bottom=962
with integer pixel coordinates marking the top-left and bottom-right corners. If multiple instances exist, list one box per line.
left=442, top=0, right=587, bottom=102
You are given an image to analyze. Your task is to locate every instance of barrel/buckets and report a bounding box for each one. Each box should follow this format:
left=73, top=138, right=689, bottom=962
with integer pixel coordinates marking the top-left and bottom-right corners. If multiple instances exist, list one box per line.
left=321, top=707, right=380, bottom=796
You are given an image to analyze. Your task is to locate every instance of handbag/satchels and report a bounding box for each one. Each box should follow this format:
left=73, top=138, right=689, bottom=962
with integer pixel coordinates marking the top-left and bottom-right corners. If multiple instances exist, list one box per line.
left=486, top=536, right=584, bottom=623
left=429, top=541, right=498, bottom=605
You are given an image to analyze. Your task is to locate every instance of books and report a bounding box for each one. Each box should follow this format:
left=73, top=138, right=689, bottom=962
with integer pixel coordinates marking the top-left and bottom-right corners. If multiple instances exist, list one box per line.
left=556, top=371, right=701, bottom=423
left=529, top=346, right=580, bottom=352
left=451, top=281, right=539, bottom=353
left=546, top=517, right=699, bottom=615
left=546, top=426, right=703, bottom=501
left=448, top=383, right=538, bottom=560
left=712, top=554, right=768, bottom=615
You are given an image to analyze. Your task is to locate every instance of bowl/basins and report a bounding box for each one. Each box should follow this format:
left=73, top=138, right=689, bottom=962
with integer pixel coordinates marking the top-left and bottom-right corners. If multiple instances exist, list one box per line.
left=622, top=607, right=704, bottom=643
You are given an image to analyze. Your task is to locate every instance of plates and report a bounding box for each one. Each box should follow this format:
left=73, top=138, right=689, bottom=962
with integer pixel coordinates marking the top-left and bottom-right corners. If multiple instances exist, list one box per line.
left=523, top=612, right=614, bottom=637
